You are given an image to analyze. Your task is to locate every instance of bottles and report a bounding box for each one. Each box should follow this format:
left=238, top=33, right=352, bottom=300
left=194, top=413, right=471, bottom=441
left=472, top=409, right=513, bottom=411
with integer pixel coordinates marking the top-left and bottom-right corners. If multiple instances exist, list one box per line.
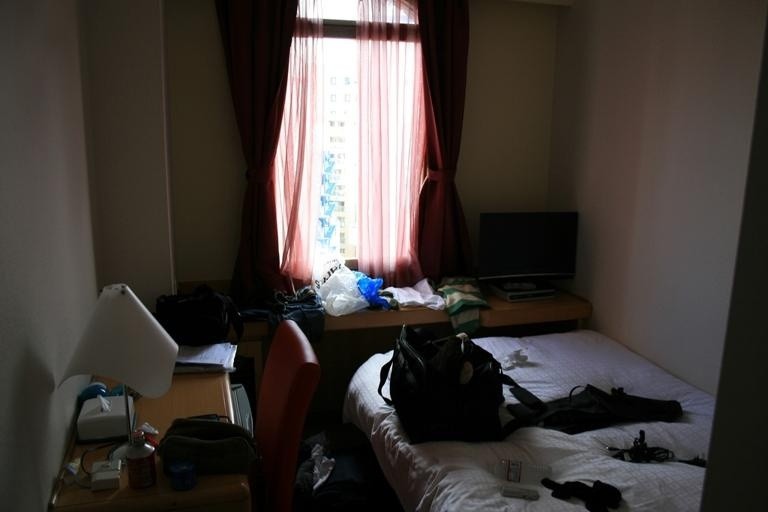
left=484, top=459, right=553, bottom=484
left=125, top=430, right=157, bottom=489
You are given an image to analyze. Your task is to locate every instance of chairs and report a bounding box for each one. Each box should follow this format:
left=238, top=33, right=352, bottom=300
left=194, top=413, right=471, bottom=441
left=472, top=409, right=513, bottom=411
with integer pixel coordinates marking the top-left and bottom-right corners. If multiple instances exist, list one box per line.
left=259, top=314, right=321, bottom=512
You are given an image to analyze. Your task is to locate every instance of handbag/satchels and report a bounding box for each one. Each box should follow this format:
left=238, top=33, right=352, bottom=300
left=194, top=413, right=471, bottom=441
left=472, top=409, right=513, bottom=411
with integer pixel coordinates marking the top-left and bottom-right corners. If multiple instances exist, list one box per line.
left=159, top=417, right=261, bottom=478
left=379, top=322, right=547, bottom=439
left=154, top=288, right=244, bottom=344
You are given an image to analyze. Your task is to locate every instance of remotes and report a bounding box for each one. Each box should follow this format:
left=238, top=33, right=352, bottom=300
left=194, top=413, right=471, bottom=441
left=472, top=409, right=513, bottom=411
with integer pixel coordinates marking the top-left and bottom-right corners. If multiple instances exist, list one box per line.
left=507, top=459, right=521, bottom=482
left=500, top=482, right=539, bottom=501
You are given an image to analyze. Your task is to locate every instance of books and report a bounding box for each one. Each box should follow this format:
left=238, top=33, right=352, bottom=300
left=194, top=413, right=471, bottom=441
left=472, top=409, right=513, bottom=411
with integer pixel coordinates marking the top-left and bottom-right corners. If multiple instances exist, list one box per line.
left=166, top=342, right=237, bottom=372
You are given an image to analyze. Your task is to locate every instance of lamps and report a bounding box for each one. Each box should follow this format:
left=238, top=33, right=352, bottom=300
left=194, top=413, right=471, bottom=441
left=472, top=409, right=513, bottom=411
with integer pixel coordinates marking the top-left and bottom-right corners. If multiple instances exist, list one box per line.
left=58, top=283, right=178, bottom=490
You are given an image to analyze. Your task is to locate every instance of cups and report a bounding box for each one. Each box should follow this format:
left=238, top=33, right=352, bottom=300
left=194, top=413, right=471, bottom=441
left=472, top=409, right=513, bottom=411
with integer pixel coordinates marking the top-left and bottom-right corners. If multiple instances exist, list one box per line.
left=168, top=464, right=197, bottom=490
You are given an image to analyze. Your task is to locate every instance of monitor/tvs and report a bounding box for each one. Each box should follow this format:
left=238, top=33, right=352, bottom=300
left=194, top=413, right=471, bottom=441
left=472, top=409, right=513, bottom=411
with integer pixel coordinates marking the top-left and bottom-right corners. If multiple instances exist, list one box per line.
left=477, top=211, right=578, bottom=288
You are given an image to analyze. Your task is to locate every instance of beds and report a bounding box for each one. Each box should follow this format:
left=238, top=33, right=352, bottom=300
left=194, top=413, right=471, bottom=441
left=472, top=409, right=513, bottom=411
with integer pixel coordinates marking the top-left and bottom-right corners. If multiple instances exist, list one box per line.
left=339, top=326, right=715, bottom=512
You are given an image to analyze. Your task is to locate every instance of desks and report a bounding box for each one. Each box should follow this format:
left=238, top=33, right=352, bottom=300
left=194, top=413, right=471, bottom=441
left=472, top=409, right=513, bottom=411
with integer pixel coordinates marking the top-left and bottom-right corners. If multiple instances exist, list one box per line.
left=175, top=284, right=592, bottom=343
left=48, top=346, right=256, bottom=511
